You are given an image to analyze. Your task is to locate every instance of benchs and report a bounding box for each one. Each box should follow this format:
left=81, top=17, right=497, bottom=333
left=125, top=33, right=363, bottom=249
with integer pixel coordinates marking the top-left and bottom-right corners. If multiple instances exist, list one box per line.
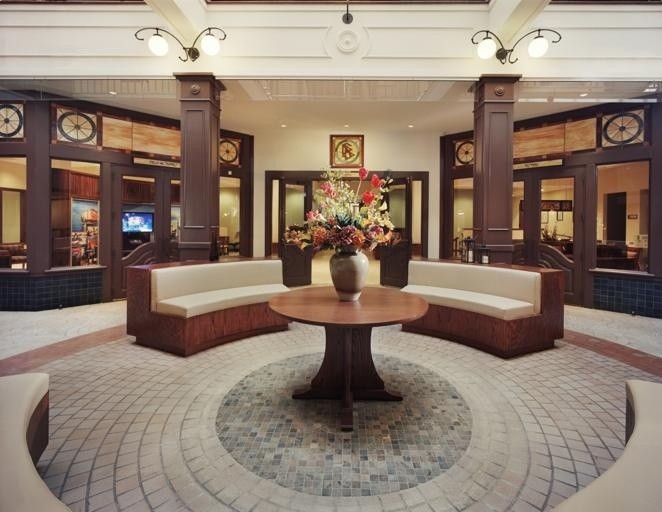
left=124, top=259, right=293, bottom=361
left=0, top=372, right=79, bottom=511
left=547, top=375, right=660, bottom=511
left=397, top=259, right=565, bottom=361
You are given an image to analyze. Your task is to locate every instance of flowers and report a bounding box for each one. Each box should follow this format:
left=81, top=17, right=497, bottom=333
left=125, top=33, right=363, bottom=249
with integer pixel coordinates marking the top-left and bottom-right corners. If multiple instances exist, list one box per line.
left=282, top=168, right=408, bottom=251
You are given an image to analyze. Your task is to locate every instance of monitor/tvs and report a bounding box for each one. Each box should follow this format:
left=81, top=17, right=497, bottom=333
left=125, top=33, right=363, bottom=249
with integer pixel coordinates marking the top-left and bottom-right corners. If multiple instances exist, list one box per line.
left=120, top=211, right=154, bottom=233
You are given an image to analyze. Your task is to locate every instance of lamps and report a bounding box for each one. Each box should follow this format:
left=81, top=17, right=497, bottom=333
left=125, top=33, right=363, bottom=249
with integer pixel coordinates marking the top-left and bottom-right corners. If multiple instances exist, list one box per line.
left=134, top=25, right=227, bottom=63
left=472, top=29, right=562, bottom=67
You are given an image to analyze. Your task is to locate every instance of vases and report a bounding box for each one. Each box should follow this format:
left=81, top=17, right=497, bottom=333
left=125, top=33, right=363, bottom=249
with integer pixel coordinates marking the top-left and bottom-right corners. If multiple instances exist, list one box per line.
left=329, top=245, right=369, bottom=302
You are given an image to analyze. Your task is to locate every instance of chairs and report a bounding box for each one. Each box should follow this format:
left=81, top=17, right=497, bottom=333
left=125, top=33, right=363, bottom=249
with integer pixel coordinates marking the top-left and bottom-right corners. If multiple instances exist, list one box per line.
left=0, top=242, right=27, bottom=269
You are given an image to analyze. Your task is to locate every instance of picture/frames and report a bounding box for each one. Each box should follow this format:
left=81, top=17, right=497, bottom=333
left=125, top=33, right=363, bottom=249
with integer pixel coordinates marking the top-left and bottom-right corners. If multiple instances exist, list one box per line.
left=330, top=135, right=364, bottom=169
